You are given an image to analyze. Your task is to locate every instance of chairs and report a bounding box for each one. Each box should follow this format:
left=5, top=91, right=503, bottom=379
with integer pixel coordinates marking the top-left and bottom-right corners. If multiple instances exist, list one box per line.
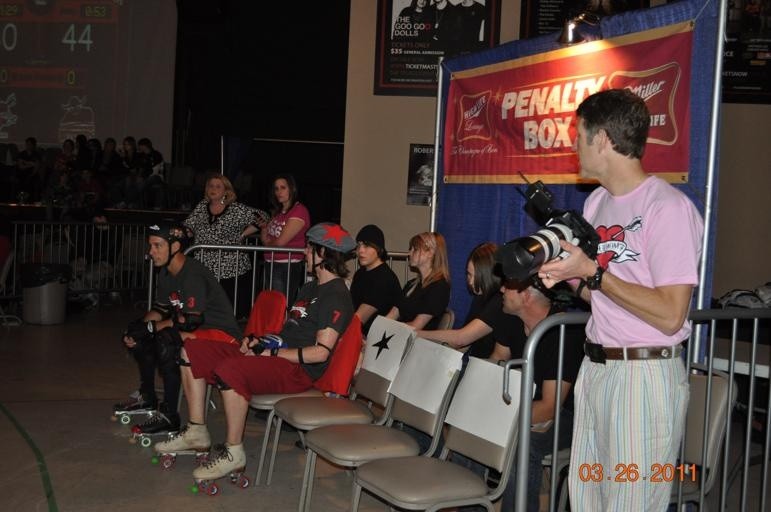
left=0, top=141, right=229, bottom=303
left=344, top=304, right=574, bottom=489
left=554, top=363, right=738, bottom=511
left=296, top=337, right=462, bottom=511
left=176, top=290, right=366, bottom=488
left=254, top=315, right=418, bottom=511
left=349, top=356, right=537, bottom=511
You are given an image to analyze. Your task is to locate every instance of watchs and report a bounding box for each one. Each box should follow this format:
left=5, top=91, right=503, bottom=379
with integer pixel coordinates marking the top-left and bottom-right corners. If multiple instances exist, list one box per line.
left=586, top=266, right=604, bottom=291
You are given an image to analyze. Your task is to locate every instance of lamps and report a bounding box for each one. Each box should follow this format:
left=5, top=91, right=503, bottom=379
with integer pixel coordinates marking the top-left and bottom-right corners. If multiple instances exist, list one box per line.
left=557, top=6, right=602, bottom=49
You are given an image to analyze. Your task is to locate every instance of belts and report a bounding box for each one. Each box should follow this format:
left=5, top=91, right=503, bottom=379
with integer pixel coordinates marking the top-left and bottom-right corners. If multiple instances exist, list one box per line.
left=582, top=339, right=682, bottom=364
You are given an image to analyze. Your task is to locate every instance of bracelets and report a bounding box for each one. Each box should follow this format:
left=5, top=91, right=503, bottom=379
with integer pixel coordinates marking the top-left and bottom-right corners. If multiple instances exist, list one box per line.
left=271, top=348, right=279, bottom=357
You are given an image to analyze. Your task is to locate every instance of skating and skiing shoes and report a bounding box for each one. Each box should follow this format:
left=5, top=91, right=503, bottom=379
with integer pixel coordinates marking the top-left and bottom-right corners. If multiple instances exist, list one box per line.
left=150, top=424, right=211, bottom=472
left=191, top=443, right=250, bottom=497
left=128, top=404, right=182, bottom=450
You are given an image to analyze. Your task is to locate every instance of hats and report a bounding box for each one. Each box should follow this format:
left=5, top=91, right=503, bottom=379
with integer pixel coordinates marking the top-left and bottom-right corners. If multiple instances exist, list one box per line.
left=356, top=224, right=385, bottom=250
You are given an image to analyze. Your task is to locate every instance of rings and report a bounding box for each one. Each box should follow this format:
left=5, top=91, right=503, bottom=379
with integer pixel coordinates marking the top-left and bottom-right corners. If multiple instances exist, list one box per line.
left=545, top=271, right=551, bottom=279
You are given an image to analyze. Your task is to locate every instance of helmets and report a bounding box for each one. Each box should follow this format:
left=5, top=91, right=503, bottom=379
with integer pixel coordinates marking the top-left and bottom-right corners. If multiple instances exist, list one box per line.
left=306, top=222, right=356, bottom=256
left=147, top=220, right=195, bottom=252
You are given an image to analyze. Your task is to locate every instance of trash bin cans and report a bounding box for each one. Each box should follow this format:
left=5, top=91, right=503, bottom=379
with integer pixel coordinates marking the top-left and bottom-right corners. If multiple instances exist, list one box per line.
left=22, top=263, right=70, bottom=325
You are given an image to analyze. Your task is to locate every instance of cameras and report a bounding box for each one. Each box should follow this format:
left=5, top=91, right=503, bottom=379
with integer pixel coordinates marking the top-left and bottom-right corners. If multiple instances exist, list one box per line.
left=503, top=180, right=600, bottom=281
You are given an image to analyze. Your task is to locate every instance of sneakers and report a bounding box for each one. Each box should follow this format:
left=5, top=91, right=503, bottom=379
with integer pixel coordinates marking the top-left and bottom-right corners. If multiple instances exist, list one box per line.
left=110, top=388, right=159, bottom=426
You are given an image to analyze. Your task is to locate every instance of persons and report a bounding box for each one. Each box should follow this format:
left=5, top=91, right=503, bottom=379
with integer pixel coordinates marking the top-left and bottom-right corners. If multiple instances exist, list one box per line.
left=349, top=223, right=402, bottom=338
left=401, top=239, right=504, bottom=460
left=112, top=220, right=244, bottom=435
left=537, top=86, right=706, bottom=512
left=393, top=0, right=485, bottom=43
left=294, top=231, right=452, bottom=449
left=451, top=235, right=593, bottom=512
left=183, top=172, right=270, bottom=321
left=0, top=133, right=169, bottom=235
left=154, top=221, right=359, bottom=482
left=258, top=172, right=311, bottom=307
